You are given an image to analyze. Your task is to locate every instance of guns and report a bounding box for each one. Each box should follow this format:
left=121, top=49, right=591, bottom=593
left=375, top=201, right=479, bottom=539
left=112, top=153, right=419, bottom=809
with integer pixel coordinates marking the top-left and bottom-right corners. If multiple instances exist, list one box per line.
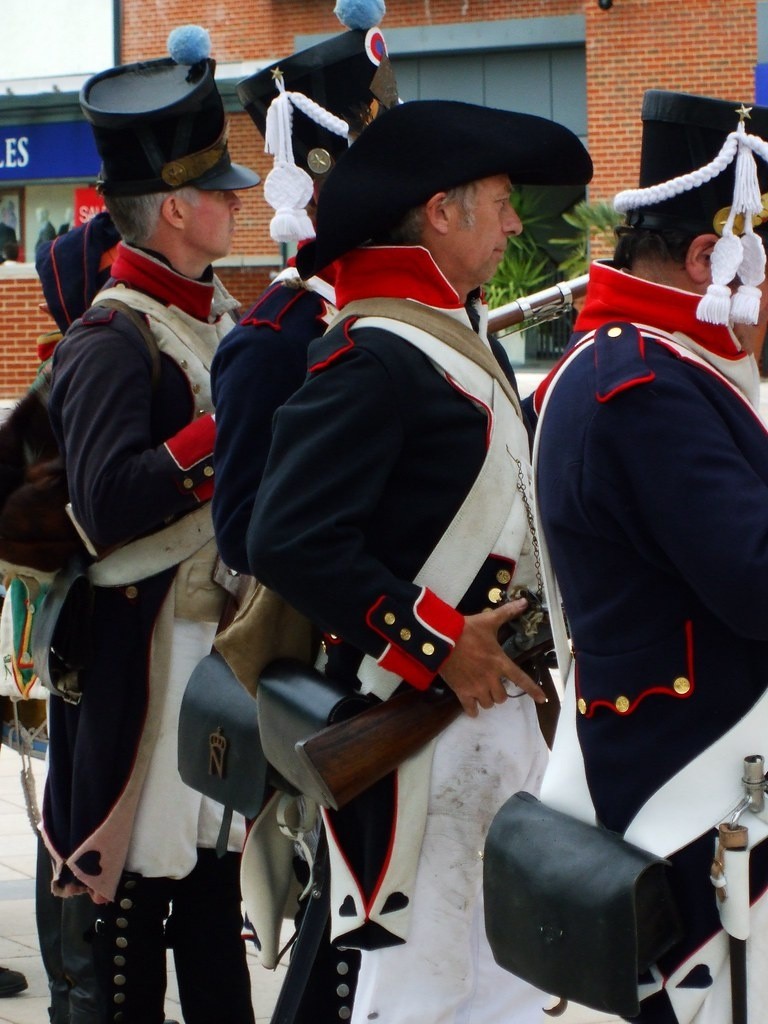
left=293, top=586, right=558, bottom=814
left=483, top=271, right=597, bottom=337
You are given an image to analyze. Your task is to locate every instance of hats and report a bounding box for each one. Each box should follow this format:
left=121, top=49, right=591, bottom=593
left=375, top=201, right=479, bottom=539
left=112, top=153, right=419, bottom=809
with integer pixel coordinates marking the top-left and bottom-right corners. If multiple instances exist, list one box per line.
left=612, top=89, right=768, bottom=326
left=295, top=99, right=594, bottom=281
left=78, top=26, right=265, bottom=195
left=235, top=0, right=396, bottom=244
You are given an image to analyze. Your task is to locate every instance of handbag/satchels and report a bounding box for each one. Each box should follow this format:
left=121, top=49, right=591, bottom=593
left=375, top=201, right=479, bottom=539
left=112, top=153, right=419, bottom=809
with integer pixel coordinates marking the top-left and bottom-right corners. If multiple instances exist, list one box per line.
left=178, top=654, right=278, bottom=821
left=259, top=655, right=355, bottom=794
left=482, top=792, right=685, bottom=1017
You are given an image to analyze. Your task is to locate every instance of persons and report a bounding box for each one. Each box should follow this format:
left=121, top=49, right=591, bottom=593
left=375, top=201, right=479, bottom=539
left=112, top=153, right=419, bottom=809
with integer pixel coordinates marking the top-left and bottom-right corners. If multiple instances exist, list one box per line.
left=243, top=99, right=592, bottom=1024
left=533, top=87, right=767, bottom=1024
left=1, top=22, right=257, bottom=1024
left=202, top=0, right=397, bottom=643
